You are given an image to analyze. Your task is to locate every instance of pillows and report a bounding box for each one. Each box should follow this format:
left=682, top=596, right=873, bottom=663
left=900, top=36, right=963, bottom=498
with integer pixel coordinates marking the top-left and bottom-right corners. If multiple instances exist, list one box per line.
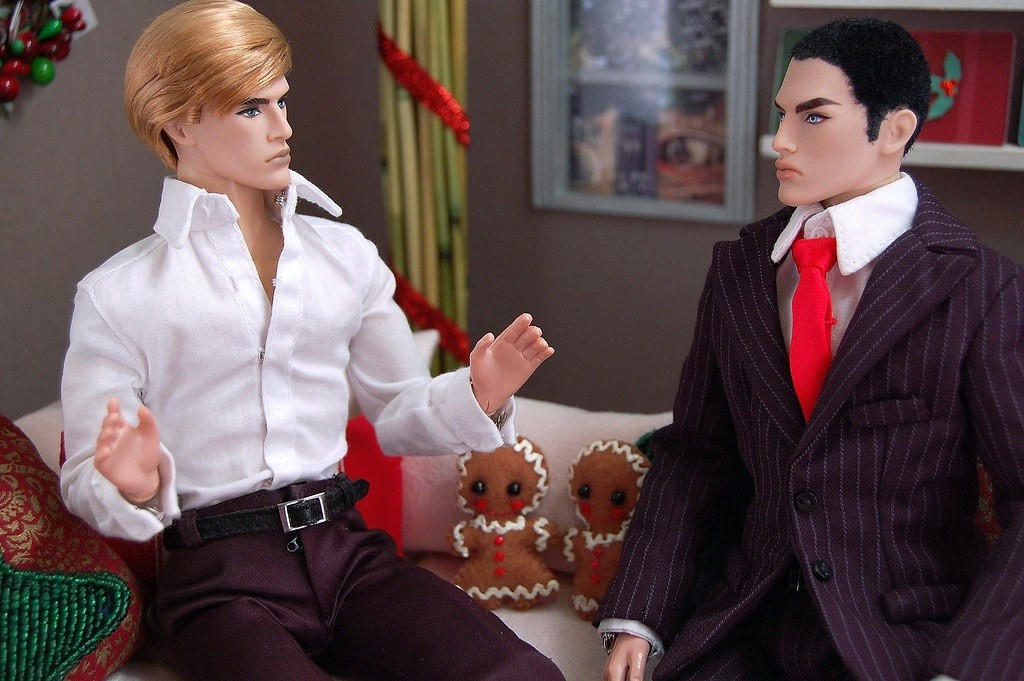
left=0, top=413, right=154, bottom=681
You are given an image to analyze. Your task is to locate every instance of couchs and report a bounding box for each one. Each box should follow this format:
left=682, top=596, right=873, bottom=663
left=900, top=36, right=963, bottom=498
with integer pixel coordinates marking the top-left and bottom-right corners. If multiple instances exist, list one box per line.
left=24, top=392, right=674, bottom=681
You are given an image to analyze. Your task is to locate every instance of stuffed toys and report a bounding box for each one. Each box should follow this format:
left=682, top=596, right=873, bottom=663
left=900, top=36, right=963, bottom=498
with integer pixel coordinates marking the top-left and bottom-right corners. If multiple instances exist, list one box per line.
left=446, top=434, right=565, bottom=610
left=561, top=438, right=651, bottom=621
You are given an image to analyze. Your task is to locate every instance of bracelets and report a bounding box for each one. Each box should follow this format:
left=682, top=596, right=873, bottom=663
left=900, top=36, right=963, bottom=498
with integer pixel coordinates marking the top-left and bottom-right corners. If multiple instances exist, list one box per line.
left=599, top=631, right=661, bottom=664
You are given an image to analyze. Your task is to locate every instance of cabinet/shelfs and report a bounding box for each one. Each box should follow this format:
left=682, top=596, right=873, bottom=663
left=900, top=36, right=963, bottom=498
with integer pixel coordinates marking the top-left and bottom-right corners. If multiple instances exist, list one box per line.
left=756, top=0, right=1024, bottom=172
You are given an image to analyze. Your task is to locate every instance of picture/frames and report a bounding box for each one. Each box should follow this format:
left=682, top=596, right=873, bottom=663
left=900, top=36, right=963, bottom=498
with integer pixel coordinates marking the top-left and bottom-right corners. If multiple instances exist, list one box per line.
left=529, top=0, right=763, bottom=225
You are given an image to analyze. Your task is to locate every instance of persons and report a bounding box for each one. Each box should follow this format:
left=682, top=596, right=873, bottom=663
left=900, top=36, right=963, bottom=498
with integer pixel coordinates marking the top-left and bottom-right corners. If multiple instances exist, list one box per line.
left=55, top=0, right=569, bottom=681
left=589, top=18, right=1024, bottom=681
